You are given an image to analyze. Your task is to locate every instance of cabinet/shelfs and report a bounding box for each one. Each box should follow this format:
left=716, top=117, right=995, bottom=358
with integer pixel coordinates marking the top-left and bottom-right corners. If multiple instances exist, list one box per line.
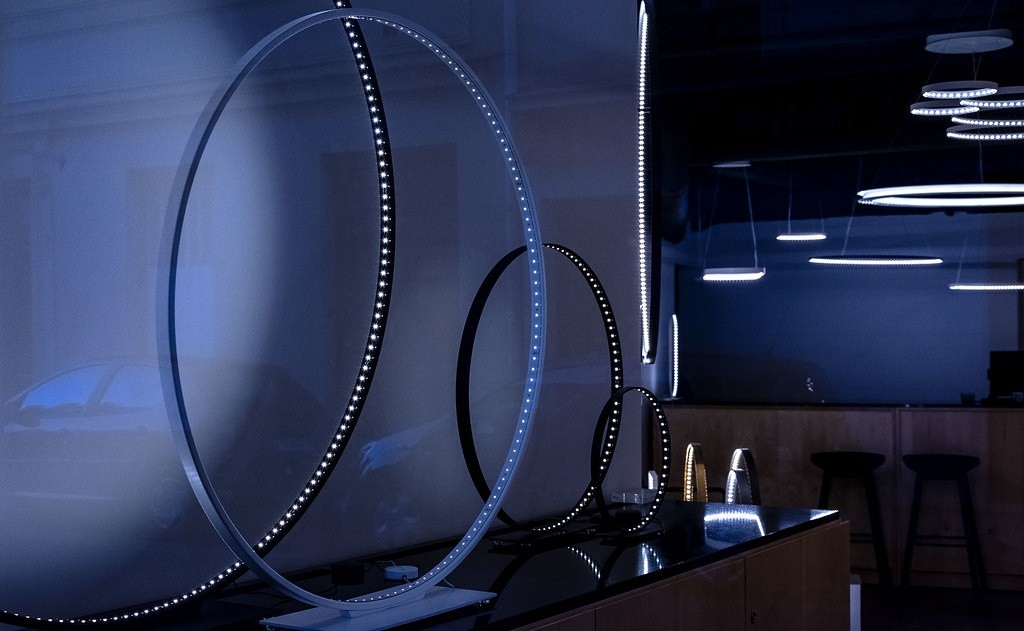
left=526, top=524, right=851, bottom=631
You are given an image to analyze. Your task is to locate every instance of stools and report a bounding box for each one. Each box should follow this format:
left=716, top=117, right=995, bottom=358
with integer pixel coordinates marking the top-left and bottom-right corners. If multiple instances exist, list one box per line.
left=809, top=452, right=891, bottom=610
left=896, top=453, right=996, bottom=611
